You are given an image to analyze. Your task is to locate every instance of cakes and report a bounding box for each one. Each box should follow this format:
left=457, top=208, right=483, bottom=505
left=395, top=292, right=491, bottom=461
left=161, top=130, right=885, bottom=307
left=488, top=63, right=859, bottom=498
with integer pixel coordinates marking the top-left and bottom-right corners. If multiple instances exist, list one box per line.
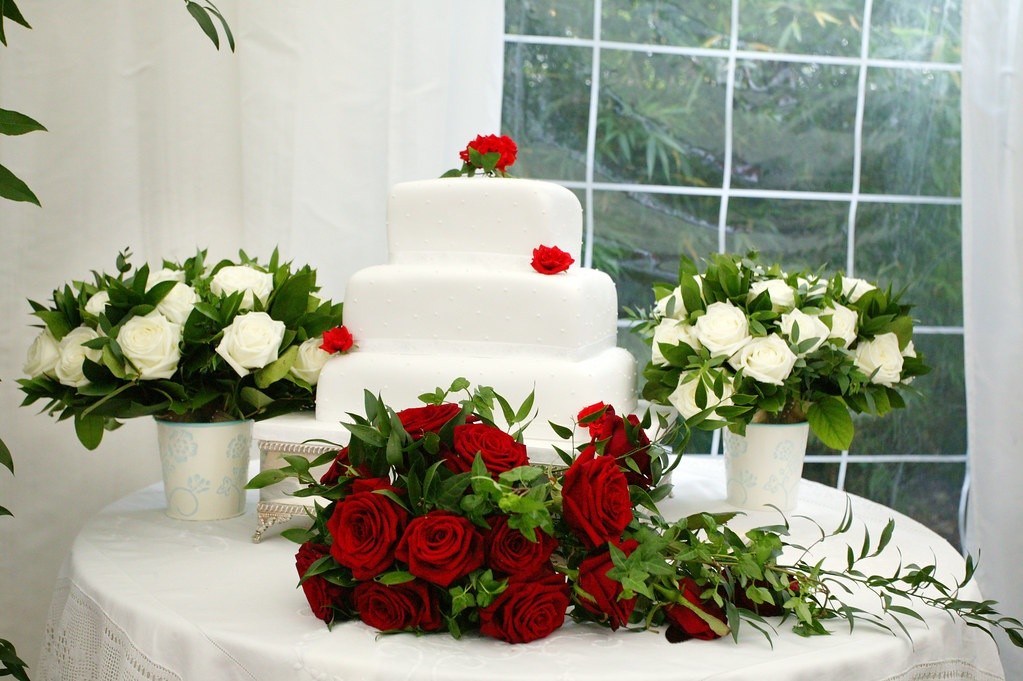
left=314, top=174, right=638, bottom=442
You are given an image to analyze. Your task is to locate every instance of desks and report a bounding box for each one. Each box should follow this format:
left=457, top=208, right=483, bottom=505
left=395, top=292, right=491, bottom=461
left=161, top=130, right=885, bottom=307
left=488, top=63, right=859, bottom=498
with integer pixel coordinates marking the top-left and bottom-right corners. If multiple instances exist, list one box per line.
left=35, top=451, right=1005, bottom=681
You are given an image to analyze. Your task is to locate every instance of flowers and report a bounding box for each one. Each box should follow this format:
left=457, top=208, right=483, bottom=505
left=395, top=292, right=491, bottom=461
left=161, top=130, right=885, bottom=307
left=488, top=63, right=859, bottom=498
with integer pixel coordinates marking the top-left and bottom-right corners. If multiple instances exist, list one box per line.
left=243, top=378, right=1023, bottom=649
left=626, top=235, right=929, bottom=449
left=14, top=246, right=357, bottom=450
left=318, top=325, right=359, bottom=356
left=530, top=244, right=577, bottom=276
left=440, top=133, right=517, bottom=180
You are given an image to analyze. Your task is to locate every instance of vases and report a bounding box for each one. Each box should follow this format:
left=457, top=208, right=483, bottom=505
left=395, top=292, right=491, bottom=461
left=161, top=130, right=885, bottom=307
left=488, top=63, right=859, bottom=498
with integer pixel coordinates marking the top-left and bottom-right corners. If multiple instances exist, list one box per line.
left=156, top=418, right=257, bottom=521
left=719, top=422, right=811, bottom=513
